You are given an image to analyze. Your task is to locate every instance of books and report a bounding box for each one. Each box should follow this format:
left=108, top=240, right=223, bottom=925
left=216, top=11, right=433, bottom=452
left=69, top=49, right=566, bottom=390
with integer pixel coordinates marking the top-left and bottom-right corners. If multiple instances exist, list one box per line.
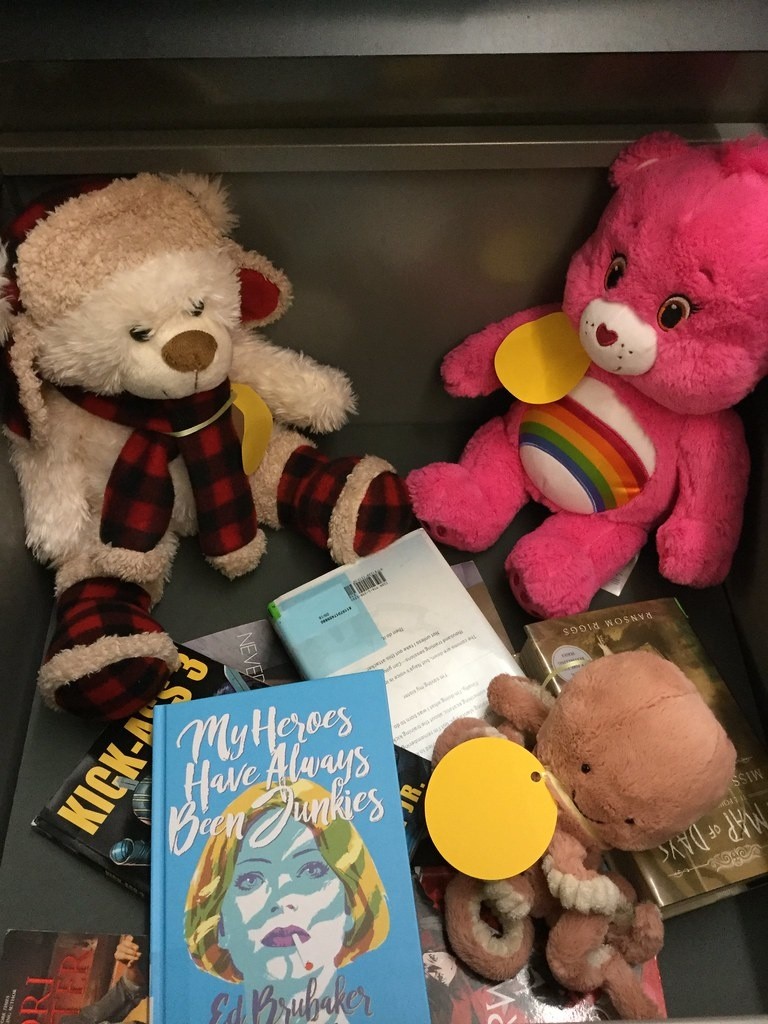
left=2, top=529, right=768, bottom=1024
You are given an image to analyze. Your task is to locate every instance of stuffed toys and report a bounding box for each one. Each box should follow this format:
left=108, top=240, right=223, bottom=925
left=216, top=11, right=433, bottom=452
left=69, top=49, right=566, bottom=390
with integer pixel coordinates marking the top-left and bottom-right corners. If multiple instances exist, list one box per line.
left=407, top=133, right=768, bottom=617
left=2, top=169, right=409, bottom=720
